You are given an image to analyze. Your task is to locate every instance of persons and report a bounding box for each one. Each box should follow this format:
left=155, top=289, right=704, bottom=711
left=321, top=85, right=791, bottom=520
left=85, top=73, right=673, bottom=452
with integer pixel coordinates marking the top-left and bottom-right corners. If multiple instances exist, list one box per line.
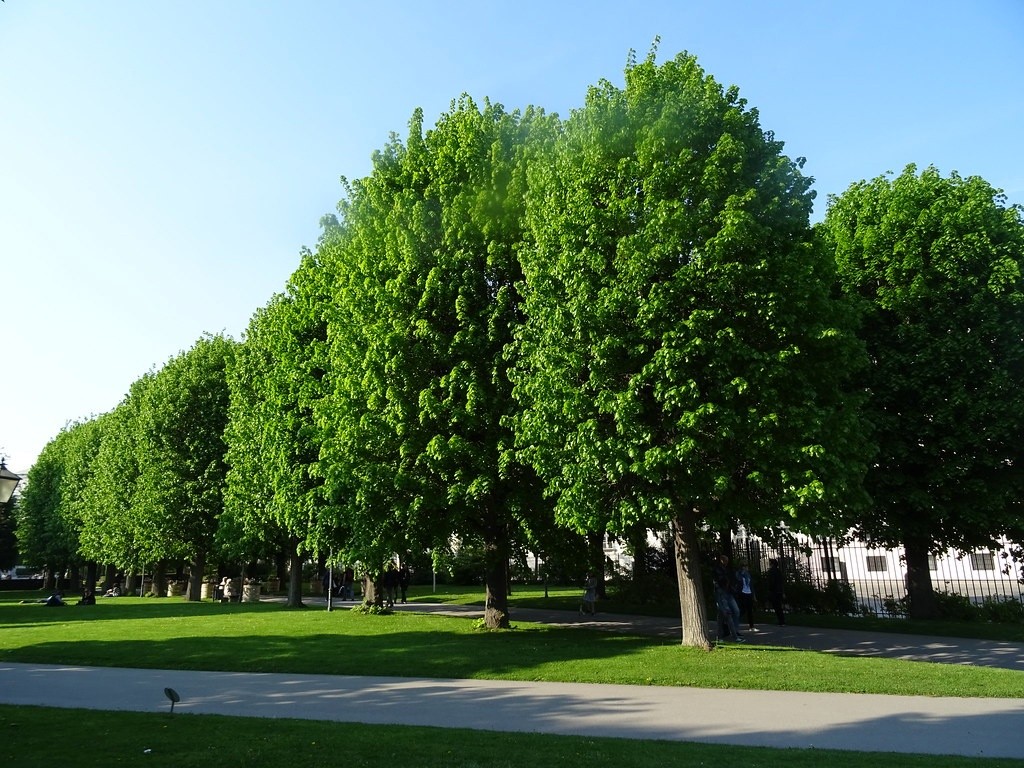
left=103, top=583, right=118, bottom=597
left=342, top=568, right=355, bottom=600
left=84, top=587, right=95, bottom=605
left=217, top=576, right=231, bottom=602
left=768, top=559, right=787, bottom=626
left=583, top=571, right=597, bottom=616
left=384, top=564, right=410, bottom=607
left=712, top=555, right=761, bottom=643
left=47, top=592, right=66, bottom=606
left=322, top=568, right=330, bottom=600
left=167, top=580, right=178, bottom=597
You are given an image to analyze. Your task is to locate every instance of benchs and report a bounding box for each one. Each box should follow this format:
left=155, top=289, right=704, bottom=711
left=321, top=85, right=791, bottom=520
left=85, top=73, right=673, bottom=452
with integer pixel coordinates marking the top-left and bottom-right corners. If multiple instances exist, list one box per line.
left=212, top=585, right=228, bottom=603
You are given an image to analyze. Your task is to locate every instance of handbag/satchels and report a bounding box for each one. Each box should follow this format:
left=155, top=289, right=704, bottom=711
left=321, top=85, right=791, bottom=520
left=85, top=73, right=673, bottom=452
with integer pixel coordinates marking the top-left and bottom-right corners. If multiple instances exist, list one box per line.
left=338, top=586, right=346, bottom=598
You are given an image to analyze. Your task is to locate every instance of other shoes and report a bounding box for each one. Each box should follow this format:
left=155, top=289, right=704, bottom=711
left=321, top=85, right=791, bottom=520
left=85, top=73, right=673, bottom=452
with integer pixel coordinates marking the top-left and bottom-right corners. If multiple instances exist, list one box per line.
left=401, top=601, right=408, bottom=604
left=734, top=638, right=746, bottom=642
left=579, top=612, right=586, bottom=616
left=592, top=613, right=596, bottom=616
left=386, top=602, right=393, bottom=608
left=748, top=628, right=761, bottom=633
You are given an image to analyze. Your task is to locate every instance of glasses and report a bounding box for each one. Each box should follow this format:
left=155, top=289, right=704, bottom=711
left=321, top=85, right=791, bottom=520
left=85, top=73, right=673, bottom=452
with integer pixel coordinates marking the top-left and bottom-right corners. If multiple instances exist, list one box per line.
left=741, top=564, right=748, bottom=567
